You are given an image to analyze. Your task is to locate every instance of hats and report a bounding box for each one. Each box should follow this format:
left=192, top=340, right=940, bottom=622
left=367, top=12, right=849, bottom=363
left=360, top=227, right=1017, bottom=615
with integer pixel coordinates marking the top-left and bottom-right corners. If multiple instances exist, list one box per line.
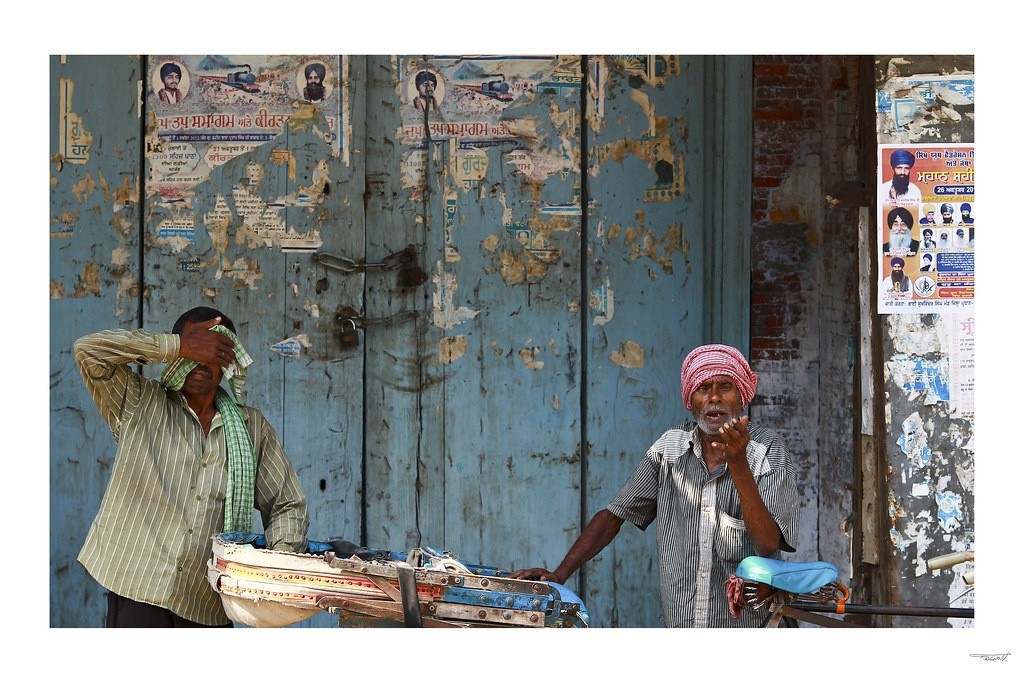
left=305, top=63, right=325, bottom=82
left=960, top=203, right=971, bottom=213
left=160, top=63, right=181, bottom=82
left=416, top=71, right=437, bottom=90
left=923, top=254, right=932, bottom=262
left=923, top=229, right=933, bottom=236
left=941, top=203, right=954, bottom=214
left=888, top=208, right=913, bottom=230
left=890, top=150, right=915, bottom=168
left=957, top=229, right=963, bottom=235
left=891, top=258, right=904, bottom=268
left=939, top=228, right=949, bottom=237
left=680, top=344, right=757, bottom=412
left=923, top=204, right=936, bottom=216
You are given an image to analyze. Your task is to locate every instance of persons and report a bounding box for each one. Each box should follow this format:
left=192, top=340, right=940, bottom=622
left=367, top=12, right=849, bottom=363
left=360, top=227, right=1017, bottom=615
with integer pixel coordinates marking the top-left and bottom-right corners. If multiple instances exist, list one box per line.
left=508, top=344, right=800, bottom=627
left=413, top=72, right=439, bottom=110
left=920, top=202, right=974, bottom=271
left=303, top=63, right=331, bottom=102
left=73, top=306, right=310, bottom=628
left=884, top=257, right=913, bottom=300
left=156, top=64, right=183, bottom=102
left=884, top=207, right=920, bottom=256
left=883, top=152, right=921, bottom=205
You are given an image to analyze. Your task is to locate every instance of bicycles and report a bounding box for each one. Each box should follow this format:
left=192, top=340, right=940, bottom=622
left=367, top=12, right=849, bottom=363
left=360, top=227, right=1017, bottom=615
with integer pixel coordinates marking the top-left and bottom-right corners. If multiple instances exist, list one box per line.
left=731, top=551, right=974, bottom=628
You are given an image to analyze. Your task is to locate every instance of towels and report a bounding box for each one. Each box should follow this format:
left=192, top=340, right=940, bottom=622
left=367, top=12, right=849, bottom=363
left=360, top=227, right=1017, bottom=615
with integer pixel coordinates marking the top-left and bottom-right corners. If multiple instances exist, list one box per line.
left=157, top=321, right=256, bottom=537
